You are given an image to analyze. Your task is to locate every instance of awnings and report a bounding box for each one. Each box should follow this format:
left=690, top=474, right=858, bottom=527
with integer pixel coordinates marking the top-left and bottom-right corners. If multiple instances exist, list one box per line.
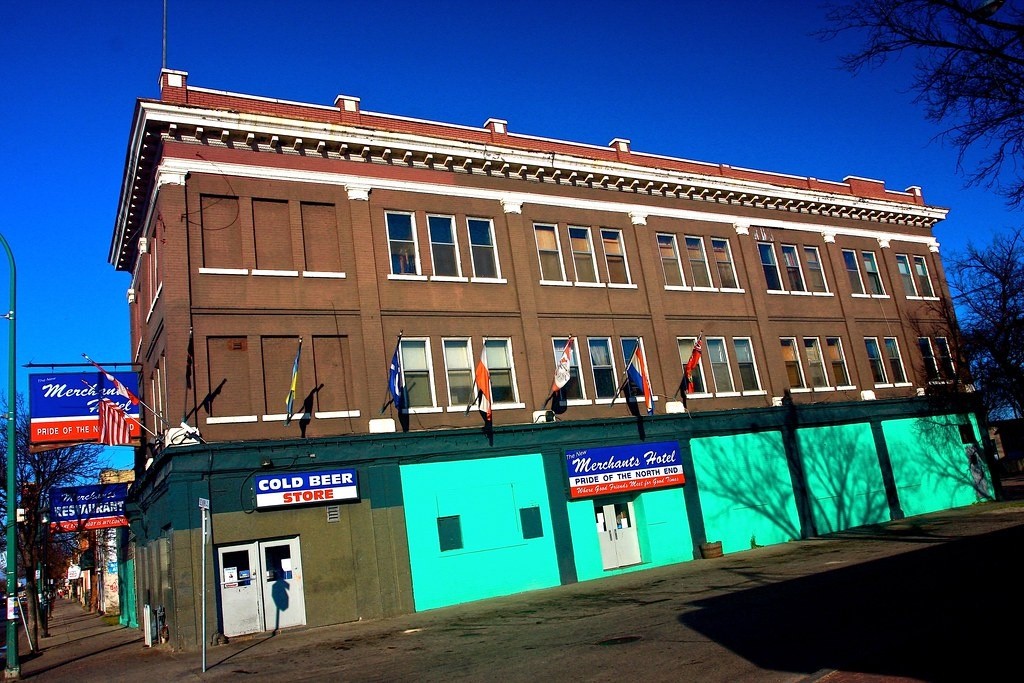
left=80, top=548, right=95, bottom=570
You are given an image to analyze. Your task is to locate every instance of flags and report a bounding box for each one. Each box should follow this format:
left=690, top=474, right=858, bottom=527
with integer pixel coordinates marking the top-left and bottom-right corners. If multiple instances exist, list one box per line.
left=388, top=346, right=401, bottom=410
left=684, top=338, right=702, bottom=394
left=91, top=361, right=139, bottom=406
left=626, top=347, right=655, bottom=416
left=549, top=346, right=570, bottom=398
left=475, top=345, right=493, bottom=421
left=97, top=393, right=131, bottom=446
left=285, top=349, right=298, bottom=414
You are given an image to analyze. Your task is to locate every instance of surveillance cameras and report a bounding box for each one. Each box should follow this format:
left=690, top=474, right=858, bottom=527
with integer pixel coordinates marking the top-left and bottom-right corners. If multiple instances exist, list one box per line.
left=180, top=421, right=196, bottom=434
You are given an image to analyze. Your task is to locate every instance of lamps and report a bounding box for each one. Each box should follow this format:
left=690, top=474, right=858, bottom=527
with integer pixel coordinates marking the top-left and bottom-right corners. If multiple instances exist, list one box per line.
left=262, top=458, right=270, bottom=465
